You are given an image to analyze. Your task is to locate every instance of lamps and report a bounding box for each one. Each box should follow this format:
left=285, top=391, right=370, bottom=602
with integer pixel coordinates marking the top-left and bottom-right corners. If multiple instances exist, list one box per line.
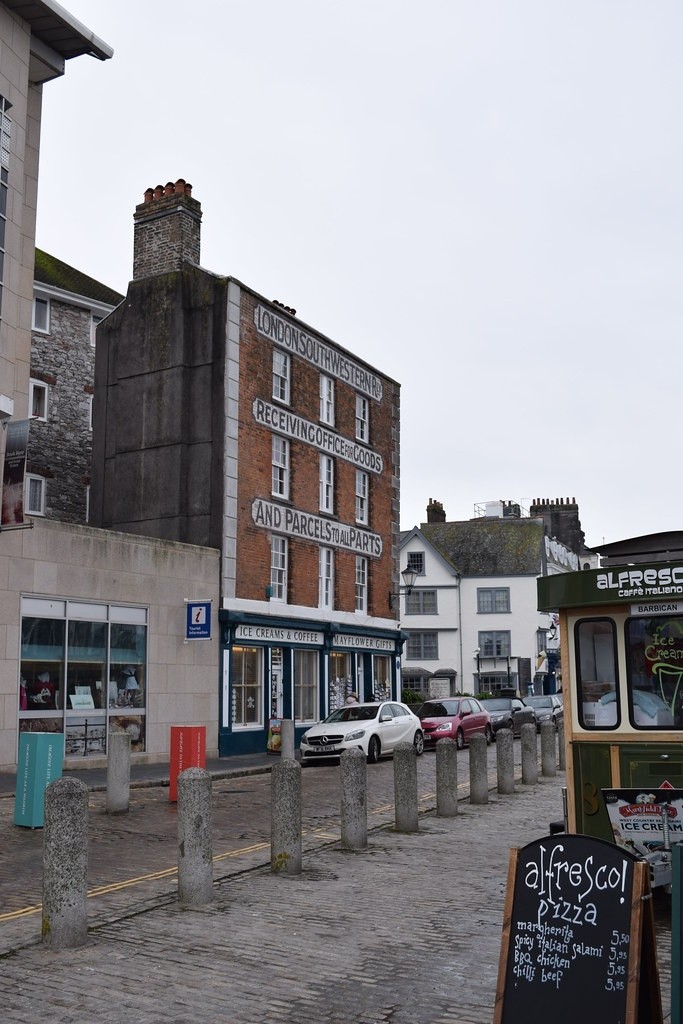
left=125, top=676, right=140, bottom=708
left=548, top=621, right=557, bottom=640
left=387, top=561, right=419, bottom=610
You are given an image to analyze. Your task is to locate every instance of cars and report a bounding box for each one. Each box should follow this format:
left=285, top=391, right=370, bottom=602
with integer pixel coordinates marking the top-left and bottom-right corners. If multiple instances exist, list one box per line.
left=474, top=694, right=538, bottom=743
left=299, top=700, right=425, bottom=766
left=413, top=696, right=492, bottom=751
left=519, top=694, right=563, bottom=734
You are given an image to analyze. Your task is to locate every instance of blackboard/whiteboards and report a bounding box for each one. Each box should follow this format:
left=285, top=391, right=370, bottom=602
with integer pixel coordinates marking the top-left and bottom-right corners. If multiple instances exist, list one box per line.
left=491, top=833, right=648, bottom=1024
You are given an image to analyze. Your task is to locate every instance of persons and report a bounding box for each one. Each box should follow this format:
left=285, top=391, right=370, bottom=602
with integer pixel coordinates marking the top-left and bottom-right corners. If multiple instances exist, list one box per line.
left=344, top=693, right=360, bottom=706
